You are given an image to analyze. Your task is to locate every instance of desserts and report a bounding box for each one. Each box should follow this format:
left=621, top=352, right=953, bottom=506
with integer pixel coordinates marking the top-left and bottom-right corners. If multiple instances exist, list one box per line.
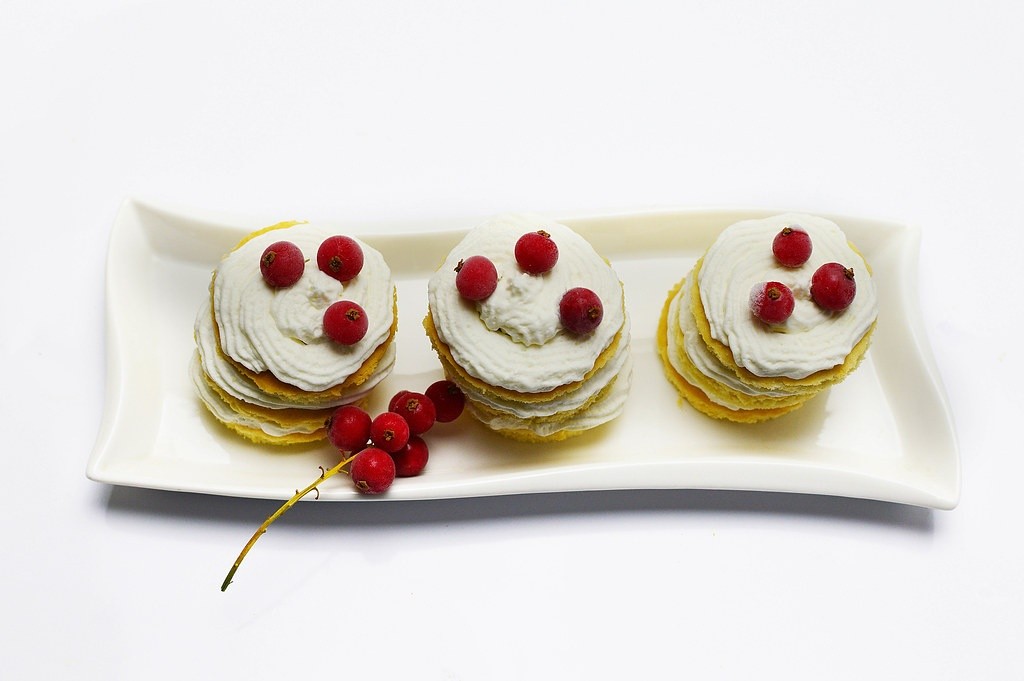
left=656, top=212, right=877, bottom=423
left=190, top=219, right=398, bottom=446
left=421, top=211, right=630, bottom=443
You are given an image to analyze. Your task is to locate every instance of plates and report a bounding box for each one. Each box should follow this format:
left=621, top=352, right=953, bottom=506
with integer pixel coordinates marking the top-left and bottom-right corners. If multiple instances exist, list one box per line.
left=86, top=194, right=961, bottom=510
